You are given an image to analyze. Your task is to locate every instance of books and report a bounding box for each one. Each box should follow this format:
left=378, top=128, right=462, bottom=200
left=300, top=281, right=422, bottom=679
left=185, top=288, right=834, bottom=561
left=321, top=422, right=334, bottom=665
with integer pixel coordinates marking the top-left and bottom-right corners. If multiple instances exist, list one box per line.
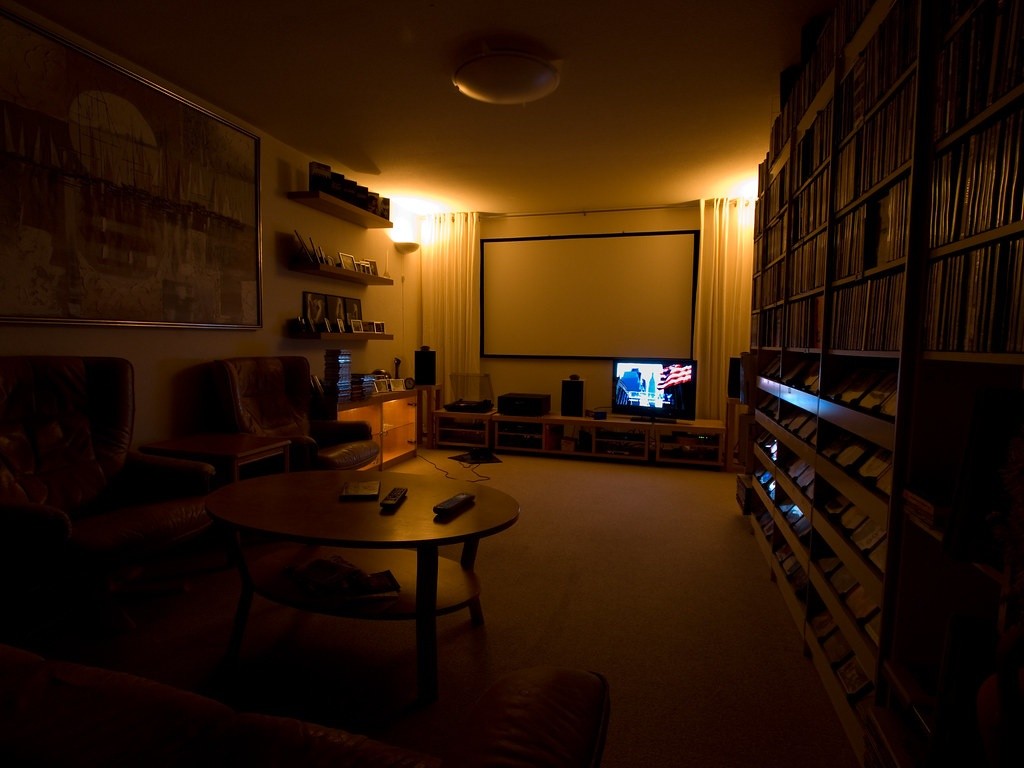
left=341, top=480, right=381, bottom=498
left=294, top=558, right=400, bottom=598
left=751, top=0, right=1024, bottom=351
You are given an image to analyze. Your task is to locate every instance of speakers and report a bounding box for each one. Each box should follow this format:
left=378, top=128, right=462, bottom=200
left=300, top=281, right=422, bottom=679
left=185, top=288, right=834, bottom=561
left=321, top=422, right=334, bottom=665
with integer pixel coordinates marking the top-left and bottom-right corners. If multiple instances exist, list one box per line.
left=728, top=357, right=742, bottom=398
left=414, top=350, right=436, bottom=385
left=561, top=379, right=583, bottom=418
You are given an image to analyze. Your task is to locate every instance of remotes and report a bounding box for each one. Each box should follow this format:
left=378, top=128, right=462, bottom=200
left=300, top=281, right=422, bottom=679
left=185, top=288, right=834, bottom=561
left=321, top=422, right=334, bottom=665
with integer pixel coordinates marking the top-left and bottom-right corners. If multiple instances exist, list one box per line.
left=380, top=488, right=408, bottom=508
left=433, top=493, right=475, bottom=516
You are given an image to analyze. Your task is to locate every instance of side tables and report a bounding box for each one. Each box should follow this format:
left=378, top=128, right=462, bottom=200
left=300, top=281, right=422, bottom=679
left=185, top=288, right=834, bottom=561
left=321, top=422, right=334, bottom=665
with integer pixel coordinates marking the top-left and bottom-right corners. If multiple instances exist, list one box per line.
left=142, top=435, right=292, bottom=486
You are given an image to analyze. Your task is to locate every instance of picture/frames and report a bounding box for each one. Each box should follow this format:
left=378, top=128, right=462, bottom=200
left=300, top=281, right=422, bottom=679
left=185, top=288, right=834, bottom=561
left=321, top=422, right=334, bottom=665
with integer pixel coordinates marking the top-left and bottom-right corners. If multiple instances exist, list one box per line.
left=302, top=291, right=385, bottom=332
left=0, top=8, right=264, bottom=333
left=294, top=228, right=380, bottom=276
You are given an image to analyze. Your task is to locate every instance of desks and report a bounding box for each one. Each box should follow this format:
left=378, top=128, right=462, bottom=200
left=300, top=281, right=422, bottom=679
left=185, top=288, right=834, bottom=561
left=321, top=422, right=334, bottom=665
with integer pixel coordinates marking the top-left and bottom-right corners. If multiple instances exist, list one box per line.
left=407, top=384, right=445, bottom=448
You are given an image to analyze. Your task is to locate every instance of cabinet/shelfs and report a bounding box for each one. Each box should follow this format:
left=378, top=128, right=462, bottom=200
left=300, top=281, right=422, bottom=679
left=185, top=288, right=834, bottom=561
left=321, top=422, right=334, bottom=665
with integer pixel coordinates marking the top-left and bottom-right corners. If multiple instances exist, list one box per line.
left=430, top=407, right=725, bottom=463
left=277, top=190, right=398, bottom=342
left=327, top=391, right=418, bottom=475
left=733, top=0, right=1024, bottom=768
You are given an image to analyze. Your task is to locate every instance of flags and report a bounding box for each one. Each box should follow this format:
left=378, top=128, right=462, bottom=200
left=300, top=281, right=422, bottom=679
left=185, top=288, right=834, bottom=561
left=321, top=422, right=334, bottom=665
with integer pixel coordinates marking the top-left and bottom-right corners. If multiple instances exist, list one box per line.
left=657, top=363, right=691, bottom=390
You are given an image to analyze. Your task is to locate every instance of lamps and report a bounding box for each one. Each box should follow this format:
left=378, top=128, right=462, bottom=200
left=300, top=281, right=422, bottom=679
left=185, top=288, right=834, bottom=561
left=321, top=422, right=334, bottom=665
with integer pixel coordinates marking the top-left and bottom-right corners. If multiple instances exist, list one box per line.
left=395, top=241, right=419, bottom=255
left=447, top=47, right=563, bottom=104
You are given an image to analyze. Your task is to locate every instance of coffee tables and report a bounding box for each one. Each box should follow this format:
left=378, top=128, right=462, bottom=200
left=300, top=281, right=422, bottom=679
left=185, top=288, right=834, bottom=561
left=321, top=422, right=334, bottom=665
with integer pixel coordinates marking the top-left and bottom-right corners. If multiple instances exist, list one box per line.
left=205, top=472, right=520, bottom=705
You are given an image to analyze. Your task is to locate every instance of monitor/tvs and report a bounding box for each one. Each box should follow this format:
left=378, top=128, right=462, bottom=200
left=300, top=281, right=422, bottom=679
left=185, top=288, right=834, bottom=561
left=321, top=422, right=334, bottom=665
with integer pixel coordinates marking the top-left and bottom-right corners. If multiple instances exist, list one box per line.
left=612, top=356, right=698, bottom=424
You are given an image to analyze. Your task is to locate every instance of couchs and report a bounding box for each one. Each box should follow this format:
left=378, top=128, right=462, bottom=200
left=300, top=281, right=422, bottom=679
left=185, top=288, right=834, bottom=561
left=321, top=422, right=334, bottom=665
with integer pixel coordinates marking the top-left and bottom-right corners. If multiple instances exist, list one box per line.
left=0, top=650, right=619, bottom=768
left=0, top=353, right=217, bottom=616
left=206, top=355, right=379, bottom=470
